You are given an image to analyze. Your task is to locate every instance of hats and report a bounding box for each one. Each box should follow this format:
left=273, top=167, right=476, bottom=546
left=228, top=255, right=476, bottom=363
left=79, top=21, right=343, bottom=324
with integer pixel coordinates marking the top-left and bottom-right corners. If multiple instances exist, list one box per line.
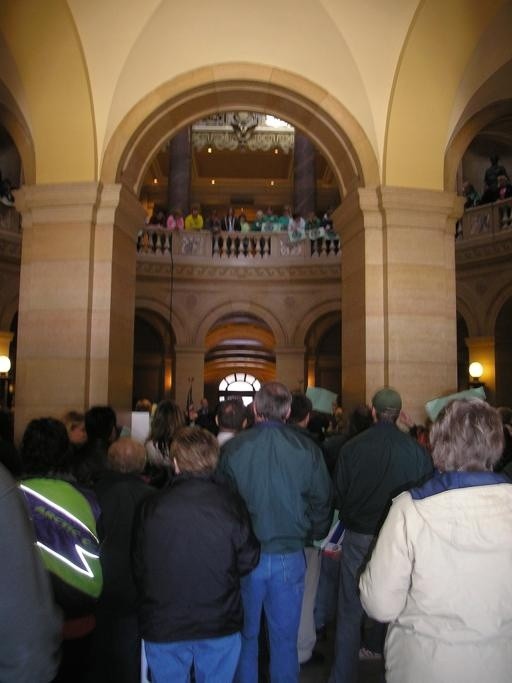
left=372, top=389, right=401, bottom=413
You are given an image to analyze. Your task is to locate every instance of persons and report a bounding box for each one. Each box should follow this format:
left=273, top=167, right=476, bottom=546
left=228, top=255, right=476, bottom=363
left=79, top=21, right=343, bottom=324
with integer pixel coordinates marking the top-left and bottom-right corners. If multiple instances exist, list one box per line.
left=136, top=205, right=341, bottom=254
left=1, top=383, right=512, bottom=683
left=485, top=154, right=507, bottom=189
left=1, top=179, right=16, bottom=218
left=462, top=175, right=511, bottom=231
